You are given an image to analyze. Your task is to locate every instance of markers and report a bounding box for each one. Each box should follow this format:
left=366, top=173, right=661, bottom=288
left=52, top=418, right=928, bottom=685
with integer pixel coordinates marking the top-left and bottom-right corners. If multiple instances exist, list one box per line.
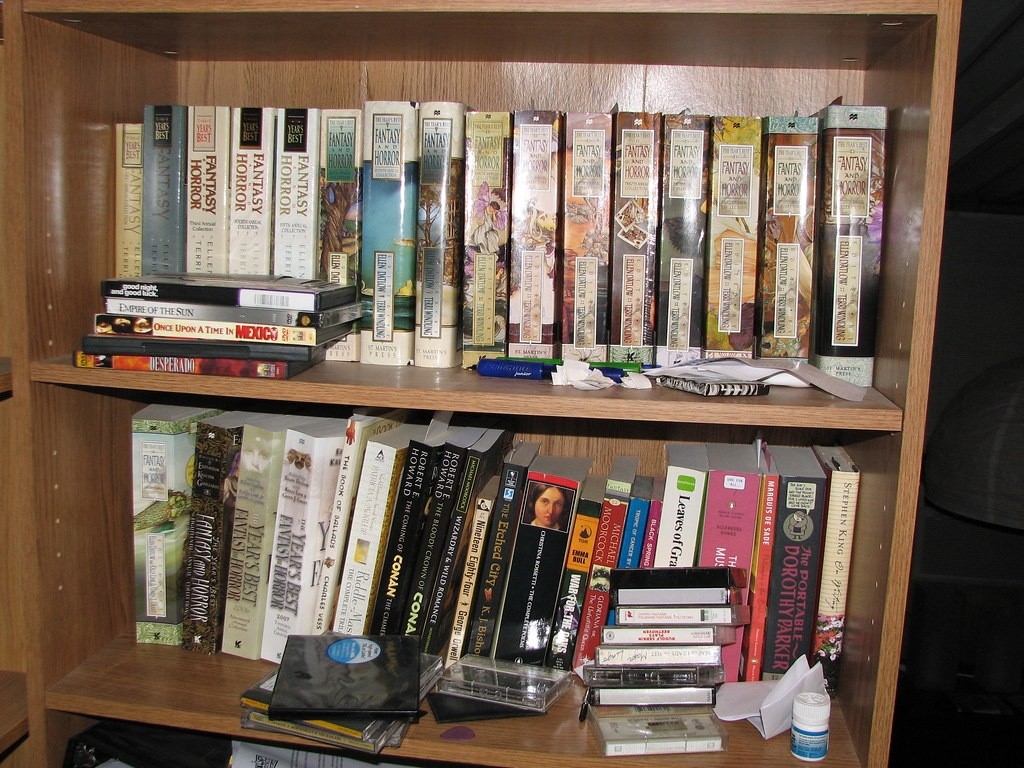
left=478, top=355, right=645, bottom=387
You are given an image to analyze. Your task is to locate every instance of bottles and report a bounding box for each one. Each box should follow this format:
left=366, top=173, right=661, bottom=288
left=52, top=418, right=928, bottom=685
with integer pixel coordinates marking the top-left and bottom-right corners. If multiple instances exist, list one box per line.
left=789, top=692, right=831, bottom=761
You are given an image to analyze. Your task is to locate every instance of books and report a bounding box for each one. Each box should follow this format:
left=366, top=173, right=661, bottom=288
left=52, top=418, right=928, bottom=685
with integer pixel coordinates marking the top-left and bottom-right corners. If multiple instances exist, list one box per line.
left=129, top=403, right=859, bottom=684
left=114, top=99, right=887, bottom=388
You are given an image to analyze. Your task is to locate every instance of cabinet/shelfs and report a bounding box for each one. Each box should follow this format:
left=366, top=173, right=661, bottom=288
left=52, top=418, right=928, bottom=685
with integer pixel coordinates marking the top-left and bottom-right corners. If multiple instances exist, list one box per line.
left=0, top=0, right=965, bottom=768
